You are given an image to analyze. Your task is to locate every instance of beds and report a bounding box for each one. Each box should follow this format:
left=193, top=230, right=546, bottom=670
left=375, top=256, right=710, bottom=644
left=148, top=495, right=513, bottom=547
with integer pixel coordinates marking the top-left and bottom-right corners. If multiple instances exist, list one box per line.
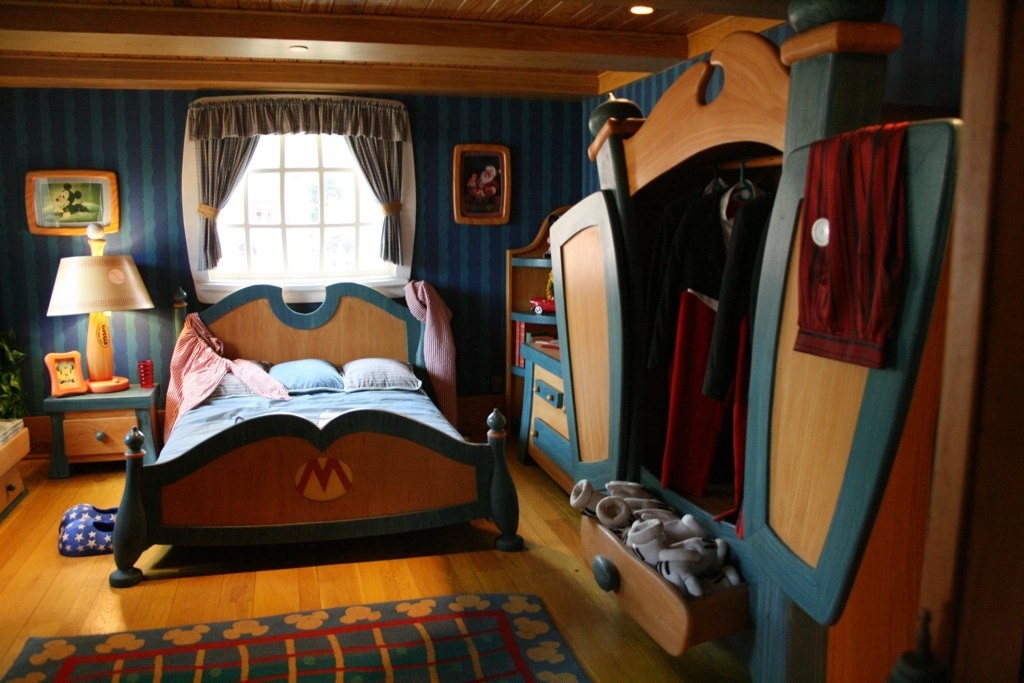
left=109, top=284, right=528, bottom=588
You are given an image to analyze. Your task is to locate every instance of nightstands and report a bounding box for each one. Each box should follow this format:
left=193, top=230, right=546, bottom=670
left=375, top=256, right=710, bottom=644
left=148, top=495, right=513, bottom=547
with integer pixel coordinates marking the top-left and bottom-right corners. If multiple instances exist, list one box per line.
left=42, top=382, right=164, bottom=480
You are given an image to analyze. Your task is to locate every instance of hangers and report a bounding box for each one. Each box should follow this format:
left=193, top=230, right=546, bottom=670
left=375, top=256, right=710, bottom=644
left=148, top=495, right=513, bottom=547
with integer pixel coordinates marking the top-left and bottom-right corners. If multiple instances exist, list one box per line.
left=708, top=156, right=755, bottom=206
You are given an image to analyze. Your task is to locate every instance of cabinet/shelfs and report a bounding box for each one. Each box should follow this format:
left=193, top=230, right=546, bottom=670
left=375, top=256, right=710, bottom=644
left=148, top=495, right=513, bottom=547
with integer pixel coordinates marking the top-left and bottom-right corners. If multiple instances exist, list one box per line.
left=500, top=204, right=573, bottom=442
left=0, top=427, right=35, bottom=521
left=542, top=33, right=960, bottom=683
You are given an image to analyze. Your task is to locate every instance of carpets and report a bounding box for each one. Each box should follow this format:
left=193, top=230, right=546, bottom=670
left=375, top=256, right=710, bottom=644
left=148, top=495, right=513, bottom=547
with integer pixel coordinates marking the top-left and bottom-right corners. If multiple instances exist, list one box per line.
left=0, top=593, right=596, bottom=683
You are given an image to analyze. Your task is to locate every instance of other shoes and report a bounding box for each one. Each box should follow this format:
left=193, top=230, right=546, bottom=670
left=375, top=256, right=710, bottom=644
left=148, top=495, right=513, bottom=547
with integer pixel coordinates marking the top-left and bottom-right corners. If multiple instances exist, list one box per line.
left=60, top=504, right=119, bottom=536
left=57, top=518, right=115, bottom=557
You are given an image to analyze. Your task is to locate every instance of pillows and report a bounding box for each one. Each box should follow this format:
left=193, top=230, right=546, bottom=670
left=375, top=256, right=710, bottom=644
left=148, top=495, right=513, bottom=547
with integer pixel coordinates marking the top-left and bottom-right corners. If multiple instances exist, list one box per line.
left=204, top=359, right=270, bottom=400
left=341, top=356, right=421, bottom=392
left=267, top=357, right=346, bottom=394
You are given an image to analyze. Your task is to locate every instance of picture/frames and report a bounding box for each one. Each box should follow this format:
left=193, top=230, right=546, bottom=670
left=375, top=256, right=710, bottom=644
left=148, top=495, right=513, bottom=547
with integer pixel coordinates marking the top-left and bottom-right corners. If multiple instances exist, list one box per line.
left=24, top=171, right=121, bottom=234
left=453, top=141, right=511, bottom=227
left=43, top=351, right=86, bottom=397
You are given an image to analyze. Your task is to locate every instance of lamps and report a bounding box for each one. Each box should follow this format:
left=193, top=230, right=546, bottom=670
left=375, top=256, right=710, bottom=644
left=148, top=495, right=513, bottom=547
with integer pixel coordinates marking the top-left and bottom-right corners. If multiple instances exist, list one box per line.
left=45, top=223, right=155, bottom=392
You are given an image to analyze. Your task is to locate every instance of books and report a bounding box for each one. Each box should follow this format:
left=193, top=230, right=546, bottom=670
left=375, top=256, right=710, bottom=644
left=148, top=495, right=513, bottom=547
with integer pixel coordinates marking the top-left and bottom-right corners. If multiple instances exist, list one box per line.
left=515, top=321, right=557, bottom=369
